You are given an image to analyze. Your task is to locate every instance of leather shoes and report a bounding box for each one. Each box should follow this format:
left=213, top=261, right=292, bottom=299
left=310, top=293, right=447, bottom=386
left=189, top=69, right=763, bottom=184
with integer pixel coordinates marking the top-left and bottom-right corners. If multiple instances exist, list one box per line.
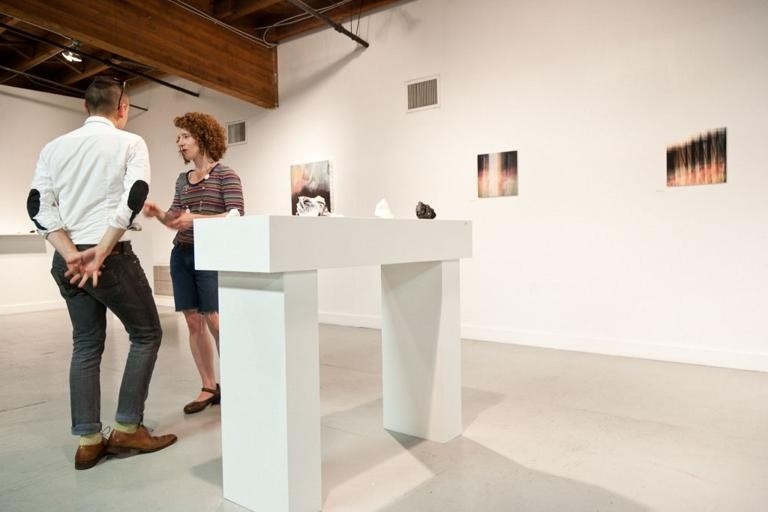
left=107, top=425, right=179, bottom=457
left=72, top=433, right=109, bottom=470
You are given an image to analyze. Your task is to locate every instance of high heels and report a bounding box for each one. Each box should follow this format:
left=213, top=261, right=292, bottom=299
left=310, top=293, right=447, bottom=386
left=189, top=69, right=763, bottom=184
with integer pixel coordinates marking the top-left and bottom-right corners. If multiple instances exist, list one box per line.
left=183, top=383, right=221, bottom=416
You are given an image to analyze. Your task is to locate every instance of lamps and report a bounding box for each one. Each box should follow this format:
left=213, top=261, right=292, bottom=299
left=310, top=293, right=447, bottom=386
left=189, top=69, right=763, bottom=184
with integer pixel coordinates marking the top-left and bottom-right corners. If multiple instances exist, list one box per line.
left=56, top=40, right=87, bottom=64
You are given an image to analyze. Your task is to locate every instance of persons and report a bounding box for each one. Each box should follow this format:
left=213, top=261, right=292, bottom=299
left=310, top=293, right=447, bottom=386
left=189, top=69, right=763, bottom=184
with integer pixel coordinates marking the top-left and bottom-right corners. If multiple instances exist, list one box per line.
left=141, top=111, right=245, bottom=413
left=27, top=74, right=178, bottom=469
